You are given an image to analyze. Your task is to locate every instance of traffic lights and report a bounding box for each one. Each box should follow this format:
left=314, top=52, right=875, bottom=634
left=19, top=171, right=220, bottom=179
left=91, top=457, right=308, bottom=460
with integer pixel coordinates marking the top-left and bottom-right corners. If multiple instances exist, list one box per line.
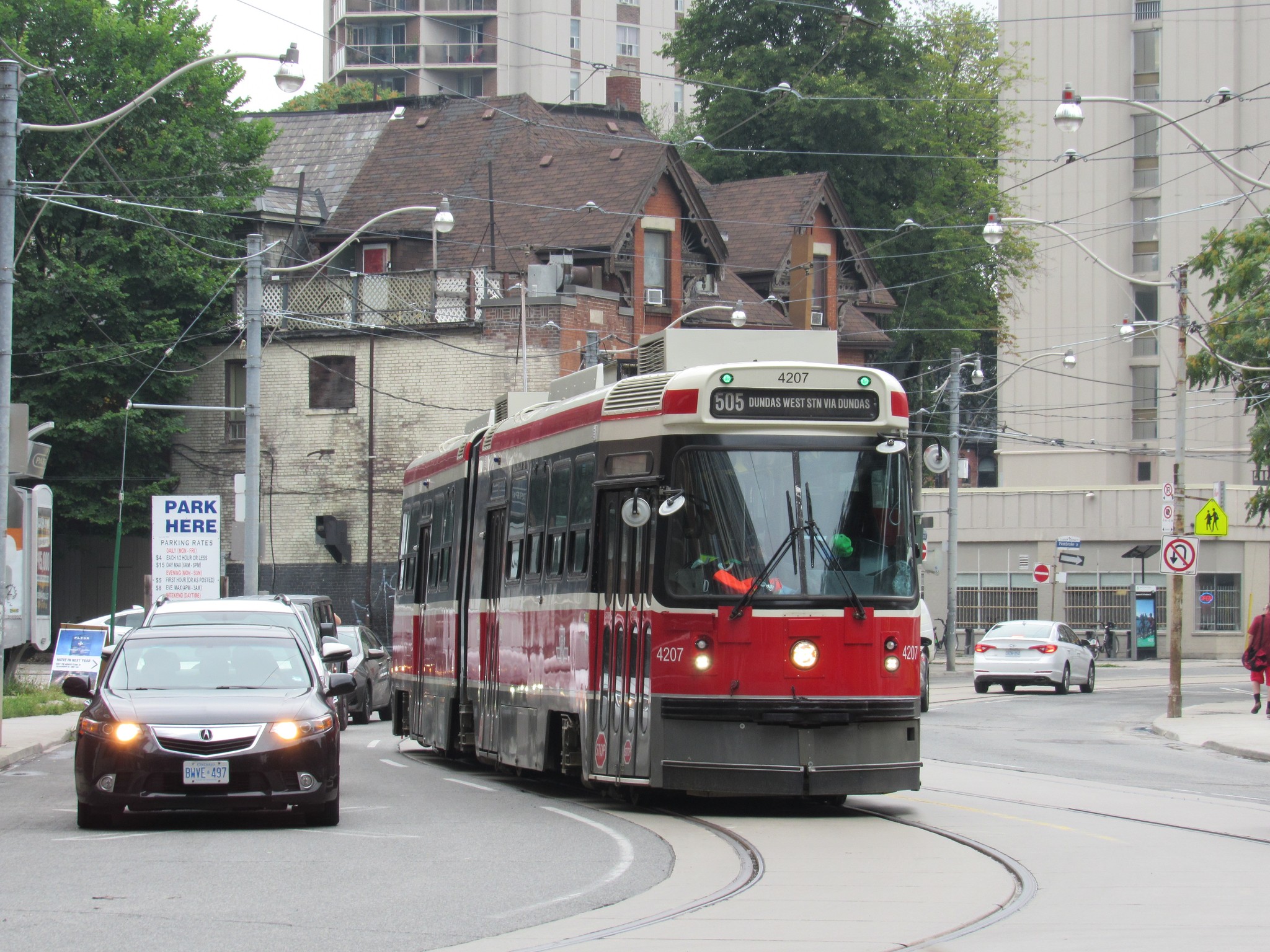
left=315, top=515, right=334, bottom=546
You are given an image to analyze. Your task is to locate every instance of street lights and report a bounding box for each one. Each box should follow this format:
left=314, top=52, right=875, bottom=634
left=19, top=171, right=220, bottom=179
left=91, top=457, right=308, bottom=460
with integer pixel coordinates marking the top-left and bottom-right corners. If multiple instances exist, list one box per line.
left=983, top=209, right=1194, bottom=719
left=583, top=301, right=746, bottom=373
left=243, top=196, right=454, bottom=600
left=946, top=347, right=1076, bottom=671
left=1, top=41, right=310, bottom=638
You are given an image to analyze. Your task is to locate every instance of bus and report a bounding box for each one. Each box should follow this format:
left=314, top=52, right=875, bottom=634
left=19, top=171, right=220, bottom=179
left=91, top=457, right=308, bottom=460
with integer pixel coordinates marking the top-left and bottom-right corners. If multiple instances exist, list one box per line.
left=390, top=320, right=951, bottom=803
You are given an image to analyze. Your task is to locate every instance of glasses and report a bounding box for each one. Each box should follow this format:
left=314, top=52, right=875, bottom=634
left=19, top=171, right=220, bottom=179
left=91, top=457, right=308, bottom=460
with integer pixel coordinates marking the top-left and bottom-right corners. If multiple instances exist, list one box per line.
left=1263, top=608, right=1268, bottom=611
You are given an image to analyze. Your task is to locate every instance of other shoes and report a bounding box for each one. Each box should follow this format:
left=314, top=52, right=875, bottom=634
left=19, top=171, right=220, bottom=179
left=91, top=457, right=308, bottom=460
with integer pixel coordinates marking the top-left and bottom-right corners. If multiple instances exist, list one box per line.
left=1266, top=708, right=1270, bottom=718
left=1251, top=702, right=1261, bottom=714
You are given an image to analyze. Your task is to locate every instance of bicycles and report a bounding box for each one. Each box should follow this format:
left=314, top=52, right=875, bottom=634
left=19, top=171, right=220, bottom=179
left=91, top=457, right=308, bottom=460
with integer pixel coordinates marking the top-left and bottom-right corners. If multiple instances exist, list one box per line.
left=1084, top=620, right=1120, bottom=660
left=932, top=618, right=959, bottom=652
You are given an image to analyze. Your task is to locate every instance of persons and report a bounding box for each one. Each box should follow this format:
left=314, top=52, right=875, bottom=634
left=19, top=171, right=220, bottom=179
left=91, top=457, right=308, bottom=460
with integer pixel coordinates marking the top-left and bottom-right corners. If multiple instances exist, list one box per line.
left=1139, top=613, right=1154, bottom=636
left=1247, top=603, right=1270, bottom=714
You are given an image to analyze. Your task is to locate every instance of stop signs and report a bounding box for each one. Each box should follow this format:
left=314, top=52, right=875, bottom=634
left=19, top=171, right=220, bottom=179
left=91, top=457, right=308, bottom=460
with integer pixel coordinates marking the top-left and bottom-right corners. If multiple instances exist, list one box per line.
left=1030, top=562, right=1051, bottom=585
left=921, top=540, right=928, bottom=562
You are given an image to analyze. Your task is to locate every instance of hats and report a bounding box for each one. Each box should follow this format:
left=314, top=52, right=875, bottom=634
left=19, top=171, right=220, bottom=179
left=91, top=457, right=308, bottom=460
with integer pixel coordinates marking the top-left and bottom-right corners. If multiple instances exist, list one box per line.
left=818, top=532, right=853, bottom=558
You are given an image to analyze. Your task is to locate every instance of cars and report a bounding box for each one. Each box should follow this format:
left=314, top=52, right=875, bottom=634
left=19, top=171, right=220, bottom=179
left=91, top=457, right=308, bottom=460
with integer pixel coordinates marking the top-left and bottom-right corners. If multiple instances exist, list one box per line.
left=334, top=625, right=393, bottom=725
left=63, top=624, right=357, bottom=832
left=972, top=620, right=1096, bottom=695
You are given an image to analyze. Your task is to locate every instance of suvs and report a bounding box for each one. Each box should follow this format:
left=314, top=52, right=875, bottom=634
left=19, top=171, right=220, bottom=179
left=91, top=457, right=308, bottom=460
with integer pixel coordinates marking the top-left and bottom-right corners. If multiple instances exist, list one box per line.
left=138, top=593, right=352, bottom=723
left=216, top=595, right=349, bottom=732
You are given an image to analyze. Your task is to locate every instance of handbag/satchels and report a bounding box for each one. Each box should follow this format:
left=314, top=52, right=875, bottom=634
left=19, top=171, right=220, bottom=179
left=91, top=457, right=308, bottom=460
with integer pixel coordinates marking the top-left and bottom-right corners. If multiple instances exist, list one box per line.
left=1241, top=646, right=1268, bottom=672
